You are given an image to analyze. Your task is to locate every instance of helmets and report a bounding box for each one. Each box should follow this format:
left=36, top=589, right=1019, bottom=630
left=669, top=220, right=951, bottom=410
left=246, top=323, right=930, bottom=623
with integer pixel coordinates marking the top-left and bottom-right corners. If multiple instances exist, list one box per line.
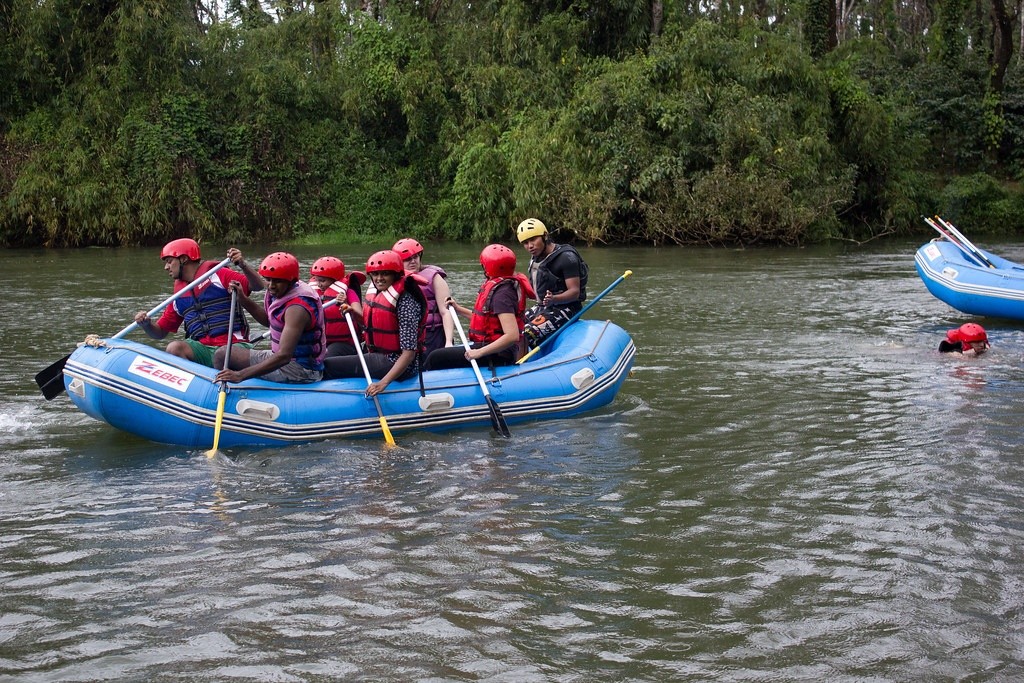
left=392, top=238, right=424, bottom=259
left=366, top=250, right=406, bottom=276
left=479, top=244, right=516, bottom=276
left=160, top=238, right=201, bottom=261
left=257, top=251, right=300, bottom=282
left=517, top=218, right=549, bottom=243
left=311, top=256, right=345, bottom=281
left=958, top=322, right=990, bottom=357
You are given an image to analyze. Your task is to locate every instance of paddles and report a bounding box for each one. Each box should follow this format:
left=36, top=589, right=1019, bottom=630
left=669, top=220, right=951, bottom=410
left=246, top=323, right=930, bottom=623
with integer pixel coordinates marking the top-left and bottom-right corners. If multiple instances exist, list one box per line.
left=204, top=282, right=240, bottom=457
left=919, top=214, right=997, bottom=270
left=445, top=297, right=513, bottom=439
left=334, top=302, right=398, bottom=447
left=35, top=251, right=239, bottom=402
left=250, top=291, right=346, bottom=344
left=516, top=270, right=633, bottom=366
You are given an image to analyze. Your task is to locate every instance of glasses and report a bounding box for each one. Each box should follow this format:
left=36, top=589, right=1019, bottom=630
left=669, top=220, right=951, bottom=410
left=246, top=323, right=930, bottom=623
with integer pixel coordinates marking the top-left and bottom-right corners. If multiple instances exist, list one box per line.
left=368, top=271, right=396, bottom=276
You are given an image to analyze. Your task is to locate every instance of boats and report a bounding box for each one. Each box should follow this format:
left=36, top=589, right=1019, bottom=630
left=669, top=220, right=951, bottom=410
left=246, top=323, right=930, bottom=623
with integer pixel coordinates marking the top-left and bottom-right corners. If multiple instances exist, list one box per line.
left=915, top=238, right=1024, bottom=321
left=63, top=319, right=633, bottom=454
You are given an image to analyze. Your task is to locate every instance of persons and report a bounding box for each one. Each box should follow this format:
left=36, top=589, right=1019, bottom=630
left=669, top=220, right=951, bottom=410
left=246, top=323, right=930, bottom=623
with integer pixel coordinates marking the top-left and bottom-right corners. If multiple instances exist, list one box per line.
left=516, top=218, right=589, bottom=354
left=423, top=243, right=527, bottom=373
left=212, top=251, right=325, bottom=385
left=392, top=238, right=454, bottom=360
left=134, top=238, right=264, bottom=369
left=321, top=249, right=429, bottom=398
left=939, top=322, right=991, bottom=359
left=307, top=257, right=364, bottom=358
